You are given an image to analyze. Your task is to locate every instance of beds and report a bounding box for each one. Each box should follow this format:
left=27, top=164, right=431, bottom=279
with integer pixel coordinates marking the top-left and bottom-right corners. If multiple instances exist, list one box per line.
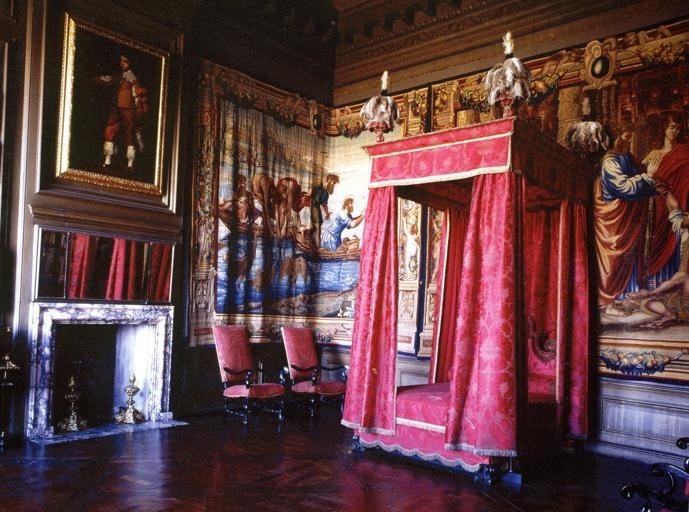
left=342, top=114, right=590, bottom=487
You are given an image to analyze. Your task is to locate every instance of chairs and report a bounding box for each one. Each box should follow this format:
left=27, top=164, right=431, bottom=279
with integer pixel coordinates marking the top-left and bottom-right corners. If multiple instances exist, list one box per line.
left=212, top=325, right=287, bottom=427
left=280, top=326, right=349, bottom=424
left=618, top=462, right=689, bottom=512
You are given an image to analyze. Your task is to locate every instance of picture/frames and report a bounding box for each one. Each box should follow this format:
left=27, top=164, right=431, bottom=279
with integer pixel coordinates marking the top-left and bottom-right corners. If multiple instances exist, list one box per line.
left=54, top=13, right=170, bottom=197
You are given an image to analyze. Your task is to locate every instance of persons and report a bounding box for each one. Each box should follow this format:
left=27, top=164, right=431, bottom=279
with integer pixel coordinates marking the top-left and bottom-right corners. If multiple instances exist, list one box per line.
left=219, top=170, right=367, bottom=253
left=594, top=114, right=689, bottom=328
left=91, top=53, right=148, bottom=173
